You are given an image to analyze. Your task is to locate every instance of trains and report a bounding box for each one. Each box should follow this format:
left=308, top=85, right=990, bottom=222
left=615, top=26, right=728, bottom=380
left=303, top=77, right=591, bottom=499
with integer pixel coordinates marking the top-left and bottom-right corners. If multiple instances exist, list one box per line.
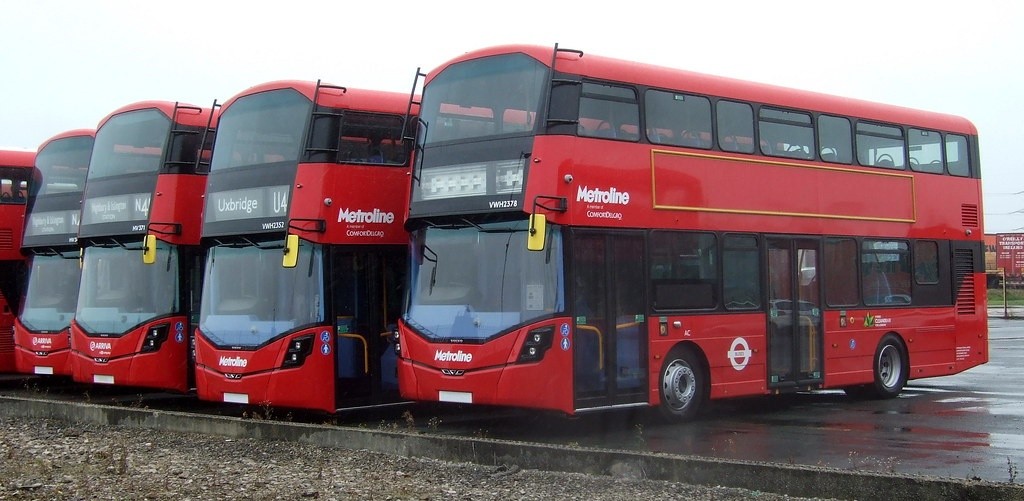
left=984, top=232, right=1024, bottom=290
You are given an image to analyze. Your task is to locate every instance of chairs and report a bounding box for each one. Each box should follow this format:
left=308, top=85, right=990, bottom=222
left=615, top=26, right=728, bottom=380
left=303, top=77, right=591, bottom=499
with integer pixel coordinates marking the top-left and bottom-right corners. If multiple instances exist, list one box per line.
left=337, top=106, right=960, bottom=199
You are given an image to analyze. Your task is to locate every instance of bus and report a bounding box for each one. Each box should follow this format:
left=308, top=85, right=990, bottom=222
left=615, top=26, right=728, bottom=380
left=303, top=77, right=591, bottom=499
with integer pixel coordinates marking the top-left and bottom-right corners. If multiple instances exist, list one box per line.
left=391, top=43, right=989, bottom=425
left=190, top=77, right=784, bottom=414
left=0, top=97, right=405, bottom=397
